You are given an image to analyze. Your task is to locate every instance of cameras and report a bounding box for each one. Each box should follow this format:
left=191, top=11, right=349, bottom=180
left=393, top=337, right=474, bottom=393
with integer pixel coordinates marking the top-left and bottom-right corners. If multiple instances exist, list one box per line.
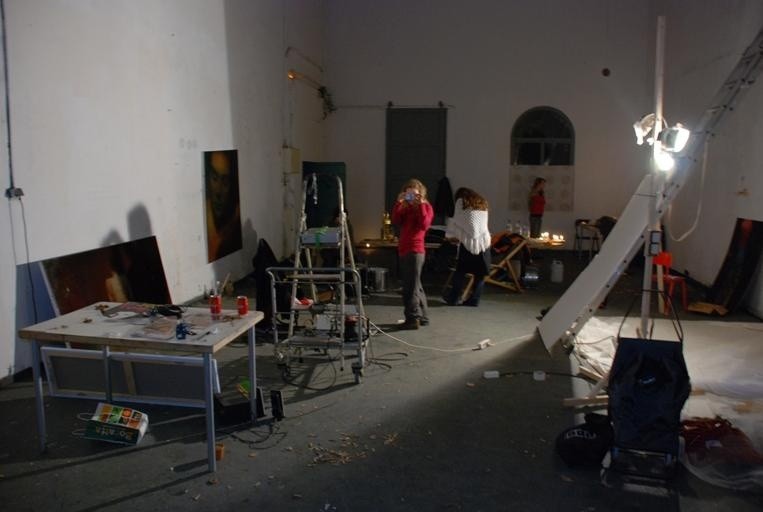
left=405, top=193, right=414, bottom=201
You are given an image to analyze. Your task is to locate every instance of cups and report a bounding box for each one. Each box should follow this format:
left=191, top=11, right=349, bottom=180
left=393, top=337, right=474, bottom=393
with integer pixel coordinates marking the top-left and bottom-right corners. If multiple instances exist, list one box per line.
left=215, top=443, right=223, bottom=460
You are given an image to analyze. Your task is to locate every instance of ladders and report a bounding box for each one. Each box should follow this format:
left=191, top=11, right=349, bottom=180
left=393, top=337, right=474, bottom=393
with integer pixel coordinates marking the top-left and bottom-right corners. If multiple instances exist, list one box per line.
left=285, top=174, right=368, bottom=371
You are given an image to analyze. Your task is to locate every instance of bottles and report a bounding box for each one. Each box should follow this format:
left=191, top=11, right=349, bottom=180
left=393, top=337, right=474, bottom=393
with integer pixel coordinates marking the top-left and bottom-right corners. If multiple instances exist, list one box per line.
left=506, top=219, right=529, bottom=239
left=384, top=220, right=392, bottom=241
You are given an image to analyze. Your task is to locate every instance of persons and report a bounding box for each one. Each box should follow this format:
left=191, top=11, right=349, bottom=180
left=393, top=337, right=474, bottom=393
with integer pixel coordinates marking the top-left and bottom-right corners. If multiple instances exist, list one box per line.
left=391, top=180, right=434, bottom=331
left=321, top=209, right=357, bottom=267
left=714, top=218, right=759, bottom=307
left=529, top=177, right=547, bottom=239
left=204, top=152, right=243, bottom=260
left=443, top=187, right=492, bottom=307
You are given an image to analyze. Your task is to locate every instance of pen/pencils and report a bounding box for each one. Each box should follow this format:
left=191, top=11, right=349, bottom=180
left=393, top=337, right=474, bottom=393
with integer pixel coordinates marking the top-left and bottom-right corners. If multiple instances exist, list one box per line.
left=197, top=332, right=210, bottom=340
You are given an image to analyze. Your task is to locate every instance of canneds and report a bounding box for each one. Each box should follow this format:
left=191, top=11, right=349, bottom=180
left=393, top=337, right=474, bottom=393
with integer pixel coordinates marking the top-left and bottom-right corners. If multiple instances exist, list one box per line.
left=236, top=296, right=248, bottom=314
left=209, top=294, right=220, bottom=314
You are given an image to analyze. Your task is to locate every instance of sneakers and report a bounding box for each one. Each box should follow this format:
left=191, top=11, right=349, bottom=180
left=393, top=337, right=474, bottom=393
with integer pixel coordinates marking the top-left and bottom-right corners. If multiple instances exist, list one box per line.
left=462, top=299, right=479, bottom=306
left=442, top=293, right=457, bottom=306
left=397, top=319, right=428, bottom=330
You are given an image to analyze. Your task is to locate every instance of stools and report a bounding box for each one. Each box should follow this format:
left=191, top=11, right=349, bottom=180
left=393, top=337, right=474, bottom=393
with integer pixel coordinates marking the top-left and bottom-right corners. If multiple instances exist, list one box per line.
left=653, top=272, right=691, bottom=316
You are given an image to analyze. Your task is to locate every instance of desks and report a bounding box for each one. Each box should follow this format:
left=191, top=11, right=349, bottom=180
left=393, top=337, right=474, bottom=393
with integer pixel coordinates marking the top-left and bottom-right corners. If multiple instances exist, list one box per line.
left=15, top=299, right=267, bottom=475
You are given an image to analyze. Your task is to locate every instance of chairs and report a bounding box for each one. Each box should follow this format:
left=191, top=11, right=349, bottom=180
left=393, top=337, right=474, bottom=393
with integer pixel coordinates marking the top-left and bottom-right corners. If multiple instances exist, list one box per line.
left=574, top=218, right=599, bottom=261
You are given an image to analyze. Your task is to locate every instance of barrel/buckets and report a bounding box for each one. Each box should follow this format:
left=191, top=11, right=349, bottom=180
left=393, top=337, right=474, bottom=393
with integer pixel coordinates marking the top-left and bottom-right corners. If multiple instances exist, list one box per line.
left=342, top=263, right=367, bottom=296
left=367, top=267, right=388, bottom=293
left=550, top=258, right=564, bottom=283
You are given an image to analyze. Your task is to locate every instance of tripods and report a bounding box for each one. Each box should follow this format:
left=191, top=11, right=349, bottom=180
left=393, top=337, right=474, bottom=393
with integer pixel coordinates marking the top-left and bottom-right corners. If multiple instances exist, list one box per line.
left=587, top=14, right=667, bottom=396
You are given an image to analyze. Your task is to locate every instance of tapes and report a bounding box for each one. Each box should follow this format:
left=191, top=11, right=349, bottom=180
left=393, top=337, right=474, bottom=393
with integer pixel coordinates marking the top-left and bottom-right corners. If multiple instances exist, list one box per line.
left=534, top=371, right=546, bottom=380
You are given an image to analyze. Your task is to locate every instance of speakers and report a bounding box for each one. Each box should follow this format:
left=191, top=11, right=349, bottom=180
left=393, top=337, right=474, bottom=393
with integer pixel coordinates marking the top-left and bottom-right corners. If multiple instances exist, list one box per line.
left=302, top=160, right=346, bottom=228
